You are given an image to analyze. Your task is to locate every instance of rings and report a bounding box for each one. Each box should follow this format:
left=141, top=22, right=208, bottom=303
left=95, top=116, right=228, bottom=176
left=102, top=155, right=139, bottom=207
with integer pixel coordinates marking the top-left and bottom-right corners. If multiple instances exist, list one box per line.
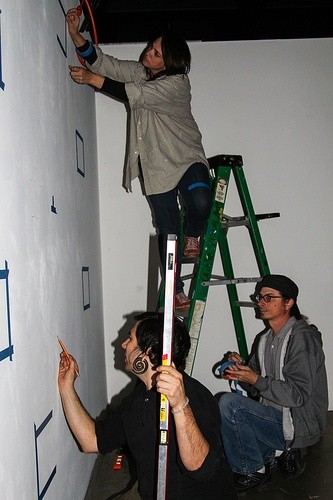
left=75, top=76, right=77, bottom=79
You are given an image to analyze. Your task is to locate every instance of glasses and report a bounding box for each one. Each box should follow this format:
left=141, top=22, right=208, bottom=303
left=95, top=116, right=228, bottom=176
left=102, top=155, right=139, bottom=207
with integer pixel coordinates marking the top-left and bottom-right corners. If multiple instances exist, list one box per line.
left=255, top=294, right=285, bottom=302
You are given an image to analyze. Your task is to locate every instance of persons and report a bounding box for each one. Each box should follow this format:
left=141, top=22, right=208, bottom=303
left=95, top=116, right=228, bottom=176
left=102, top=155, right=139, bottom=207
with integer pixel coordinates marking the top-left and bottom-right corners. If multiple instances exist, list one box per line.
left=58, top=313, right=243, bottom=500
left=217, top=274, right=328, bottom=494
left=65, top=8, right=213, bottom=311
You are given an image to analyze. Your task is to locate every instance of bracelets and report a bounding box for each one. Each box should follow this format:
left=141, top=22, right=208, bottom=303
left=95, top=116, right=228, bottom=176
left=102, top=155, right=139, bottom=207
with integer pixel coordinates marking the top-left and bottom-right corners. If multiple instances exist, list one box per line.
left=170, top=397, right=189, bottom=414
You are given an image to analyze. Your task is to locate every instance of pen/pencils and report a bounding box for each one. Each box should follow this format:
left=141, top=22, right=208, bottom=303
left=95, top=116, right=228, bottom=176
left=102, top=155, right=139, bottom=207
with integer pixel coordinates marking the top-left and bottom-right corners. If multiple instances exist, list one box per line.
left=56, top=335, right=81, bottom=378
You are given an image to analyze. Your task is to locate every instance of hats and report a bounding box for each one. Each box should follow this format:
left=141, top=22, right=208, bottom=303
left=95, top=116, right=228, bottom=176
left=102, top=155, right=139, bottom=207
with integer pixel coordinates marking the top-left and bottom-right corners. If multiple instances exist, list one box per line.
left=262, top=274, right=298, bottom=301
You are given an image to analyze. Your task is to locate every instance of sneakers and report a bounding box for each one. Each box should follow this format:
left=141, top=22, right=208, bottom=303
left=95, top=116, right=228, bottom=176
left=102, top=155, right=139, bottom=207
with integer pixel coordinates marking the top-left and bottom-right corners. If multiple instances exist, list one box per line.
left=182, top=235, right=200, bottom=259
left=174, top=293, right=191, bottom=310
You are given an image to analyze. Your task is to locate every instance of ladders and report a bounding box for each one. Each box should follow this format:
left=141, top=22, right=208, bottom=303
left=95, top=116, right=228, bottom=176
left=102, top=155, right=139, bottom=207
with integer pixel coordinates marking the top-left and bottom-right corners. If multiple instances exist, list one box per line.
left=111, top=153, right=280, bottom=472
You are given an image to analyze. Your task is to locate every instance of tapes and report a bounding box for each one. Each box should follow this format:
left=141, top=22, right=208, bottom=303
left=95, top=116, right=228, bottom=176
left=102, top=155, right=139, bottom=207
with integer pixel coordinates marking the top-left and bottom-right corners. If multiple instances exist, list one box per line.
left=219, top=361, right=237, bottom=381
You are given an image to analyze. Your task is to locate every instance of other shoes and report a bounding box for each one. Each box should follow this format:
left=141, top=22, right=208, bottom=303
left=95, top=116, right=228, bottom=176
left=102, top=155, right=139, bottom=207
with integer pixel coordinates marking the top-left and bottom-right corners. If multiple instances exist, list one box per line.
left=235, top=464, right=271, bottom=495
left=283, top=449, right=307, bottom=479
